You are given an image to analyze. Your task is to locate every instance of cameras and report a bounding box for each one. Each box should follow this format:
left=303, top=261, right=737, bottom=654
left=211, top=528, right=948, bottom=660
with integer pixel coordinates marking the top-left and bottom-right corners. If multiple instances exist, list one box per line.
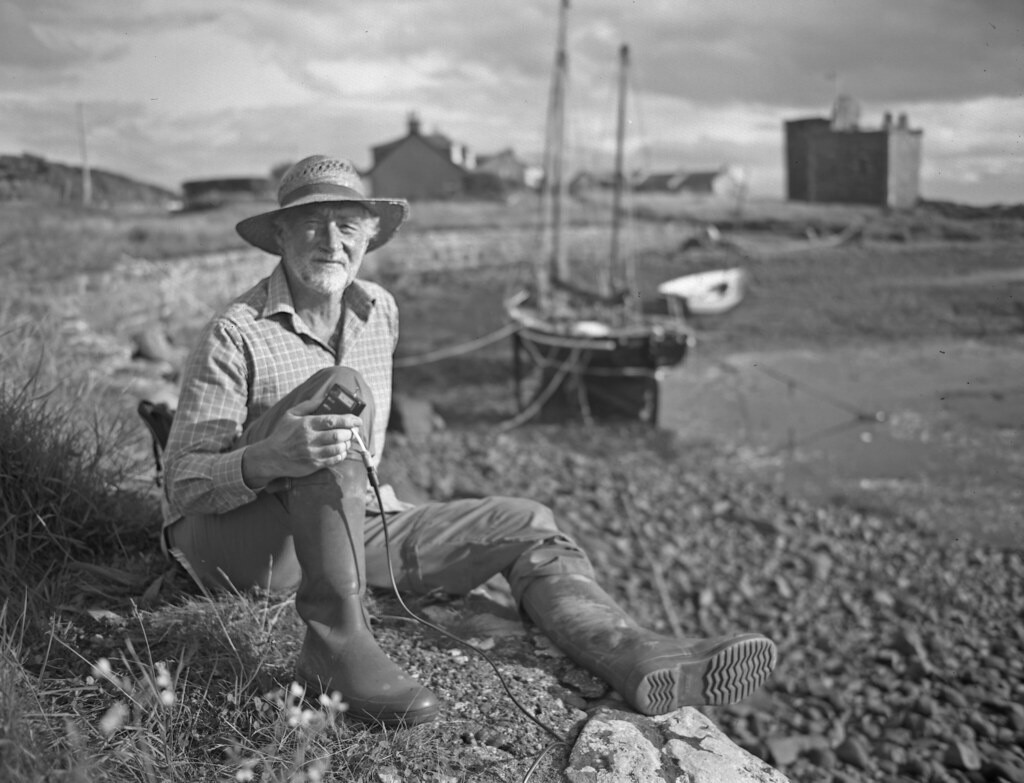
left=312, top=384, right=367, bottom=417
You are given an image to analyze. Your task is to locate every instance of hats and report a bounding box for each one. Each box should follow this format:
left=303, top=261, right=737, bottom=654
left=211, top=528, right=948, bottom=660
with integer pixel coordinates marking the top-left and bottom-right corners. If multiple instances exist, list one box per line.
left=236, top=156, right=410, bottom=256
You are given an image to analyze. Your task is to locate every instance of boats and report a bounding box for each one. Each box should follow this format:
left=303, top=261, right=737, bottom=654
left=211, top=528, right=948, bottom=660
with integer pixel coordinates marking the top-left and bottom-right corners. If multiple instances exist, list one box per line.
left=654, top=267, right=751, bottom=319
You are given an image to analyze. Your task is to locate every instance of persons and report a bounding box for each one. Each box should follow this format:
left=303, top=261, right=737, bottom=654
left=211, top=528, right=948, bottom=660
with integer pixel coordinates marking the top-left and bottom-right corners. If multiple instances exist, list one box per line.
left=160, top=153, right=780, bottom=727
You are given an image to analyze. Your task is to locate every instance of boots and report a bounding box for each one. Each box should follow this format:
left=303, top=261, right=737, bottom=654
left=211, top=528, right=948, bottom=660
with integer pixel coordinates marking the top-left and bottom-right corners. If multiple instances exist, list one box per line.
left=520, top=573, right=779, bottom=716
left=288, top=461, right=439, bottom=724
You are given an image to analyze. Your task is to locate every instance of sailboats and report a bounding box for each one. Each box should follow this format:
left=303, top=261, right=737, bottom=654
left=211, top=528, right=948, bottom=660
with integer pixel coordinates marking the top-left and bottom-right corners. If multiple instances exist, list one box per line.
left=501, top=2, right=700, bottom=376
